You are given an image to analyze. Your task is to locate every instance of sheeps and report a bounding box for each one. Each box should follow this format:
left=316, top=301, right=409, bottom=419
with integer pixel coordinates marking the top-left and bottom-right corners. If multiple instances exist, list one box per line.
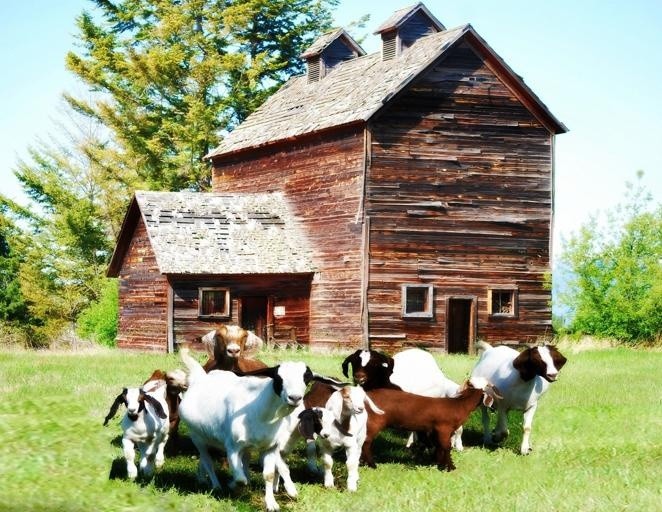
left=366, top=385, right=501, bottom=471
left=105, top=372, right=174, bottom=478
left=179, top=323, right=398, bottom=505
left=393, top=347, right=463, bottom=457
left=472, top=342, right=567, bottom=456
